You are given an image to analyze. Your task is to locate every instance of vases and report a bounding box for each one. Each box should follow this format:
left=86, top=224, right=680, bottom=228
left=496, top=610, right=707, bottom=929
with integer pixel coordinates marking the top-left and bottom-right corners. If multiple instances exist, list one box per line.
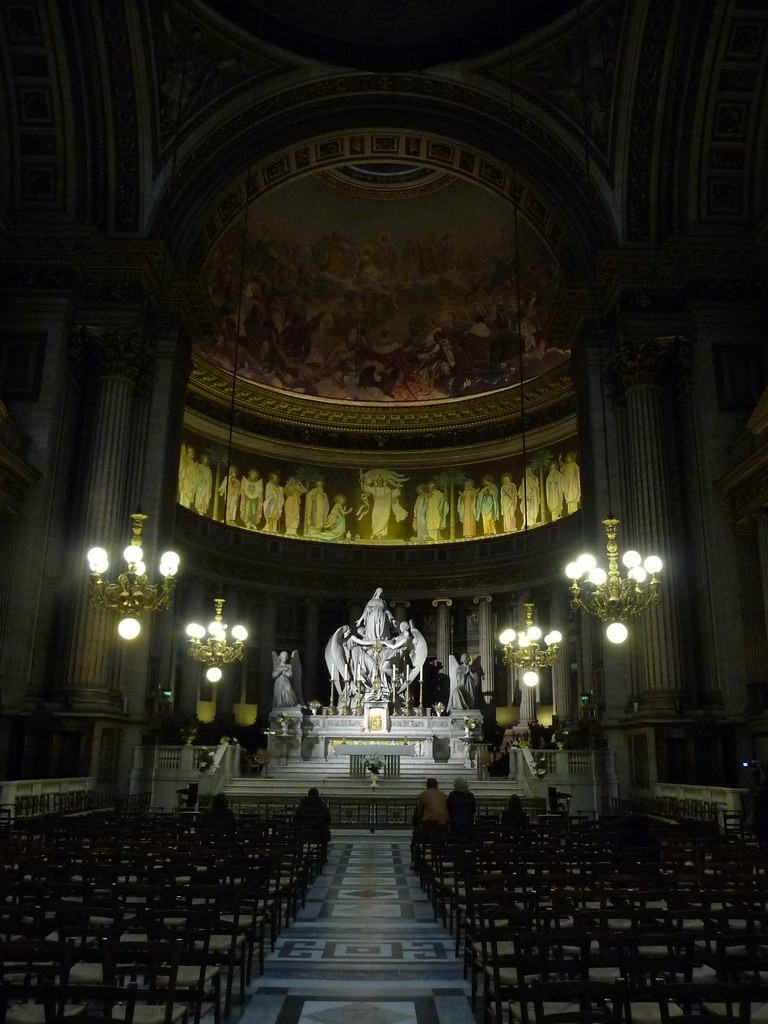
left=437, top=710, right=441, bottom=717
left=187, top=739, right=192, bottom=744
left=282, top=724, right=288, bottom=735
left=464, top=727, right=470, bottom=737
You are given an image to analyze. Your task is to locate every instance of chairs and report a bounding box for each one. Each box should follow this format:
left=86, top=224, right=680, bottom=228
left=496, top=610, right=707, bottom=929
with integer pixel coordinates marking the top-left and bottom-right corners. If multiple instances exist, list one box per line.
left=0, top=802, right=327, bottom=1024
left=411, top=813, right=768, bottom=1024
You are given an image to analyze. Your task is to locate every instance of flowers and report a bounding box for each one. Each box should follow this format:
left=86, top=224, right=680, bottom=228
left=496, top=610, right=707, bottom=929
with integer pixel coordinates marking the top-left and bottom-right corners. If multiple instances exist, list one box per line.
left=462, top=717, right=475, bottom=728
left=275, top=712, right=289, bottom=727
left=180, top=726, right=197, bottom=739
left=433, top=702, right=445, bottom=712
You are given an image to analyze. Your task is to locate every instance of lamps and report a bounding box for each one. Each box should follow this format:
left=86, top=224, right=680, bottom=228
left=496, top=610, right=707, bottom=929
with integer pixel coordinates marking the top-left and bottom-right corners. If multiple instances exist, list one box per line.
left=565, top=247, right=663, bottom=645
left=499, top=169, right=563, bottom=688
left=89, top=29, right=194, bottom=641
left=185, top=142, right=248, bottom=683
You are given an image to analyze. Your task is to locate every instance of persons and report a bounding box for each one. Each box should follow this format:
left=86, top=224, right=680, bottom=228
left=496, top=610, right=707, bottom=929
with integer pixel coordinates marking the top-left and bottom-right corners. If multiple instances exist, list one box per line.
left=293, top=788, right=331, bottom=863
left=342, top=588, right=410, bottom=686
left=272, top=651, right=297, bottom=706
left=500, top=793, right=527, bottom=827
left=452, top=654, right=476, bottom=710
left=203, top=792, right=235, bottom=830
left=447, top=776, right=476, bottom=825
left=415, top=777, right=450, bottom=827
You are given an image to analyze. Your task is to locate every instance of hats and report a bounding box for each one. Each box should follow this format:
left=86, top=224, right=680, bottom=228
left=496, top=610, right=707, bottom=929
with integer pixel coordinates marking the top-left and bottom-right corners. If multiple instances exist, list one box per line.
left=453, top=776, right=469, bottom=794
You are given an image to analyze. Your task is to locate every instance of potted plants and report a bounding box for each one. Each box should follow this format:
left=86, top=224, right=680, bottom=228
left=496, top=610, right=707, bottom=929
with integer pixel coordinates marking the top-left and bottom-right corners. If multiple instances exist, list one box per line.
left=221, top=736, right=230, bottom=745
